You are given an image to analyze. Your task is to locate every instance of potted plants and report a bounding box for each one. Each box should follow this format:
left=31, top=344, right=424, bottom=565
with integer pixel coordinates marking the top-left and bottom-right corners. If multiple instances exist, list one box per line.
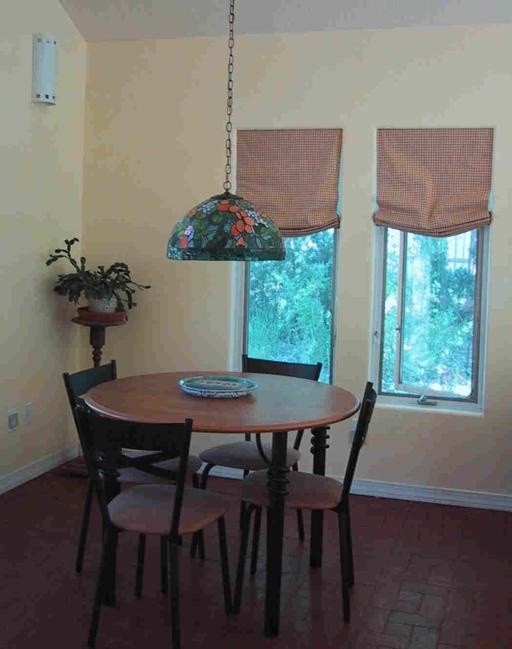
left=41, top=234, right=152, bottom=312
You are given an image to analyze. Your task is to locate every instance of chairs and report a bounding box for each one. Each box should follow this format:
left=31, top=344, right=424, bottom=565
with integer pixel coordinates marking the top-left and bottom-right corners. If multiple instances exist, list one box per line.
left=197, top=353, right=324, bottom=561
left=232, top=379, right=378, bottom=626
left=61, top=359, right=203, bottom=577
left=69, top=392, right=233, bottom=648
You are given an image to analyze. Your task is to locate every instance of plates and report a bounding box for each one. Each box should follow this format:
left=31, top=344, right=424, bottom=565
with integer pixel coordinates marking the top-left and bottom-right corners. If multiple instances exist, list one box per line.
left=175, top=373, right=259, bottom=398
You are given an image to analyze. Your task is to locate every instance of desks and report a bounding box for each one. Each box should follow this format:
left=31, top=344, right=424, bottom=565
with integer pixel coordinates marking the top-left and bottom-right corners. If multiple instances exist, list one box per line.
left=69, top=316, right=129, bottom=367
left=76, top=370, right=363, bottom=649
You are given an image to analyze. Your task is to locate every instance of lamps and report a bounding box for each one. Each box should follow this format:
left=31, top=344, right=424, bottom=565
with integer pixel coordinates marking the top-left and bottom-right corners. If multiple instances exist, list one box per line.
left=163, top=0, right=293, bottom=264
